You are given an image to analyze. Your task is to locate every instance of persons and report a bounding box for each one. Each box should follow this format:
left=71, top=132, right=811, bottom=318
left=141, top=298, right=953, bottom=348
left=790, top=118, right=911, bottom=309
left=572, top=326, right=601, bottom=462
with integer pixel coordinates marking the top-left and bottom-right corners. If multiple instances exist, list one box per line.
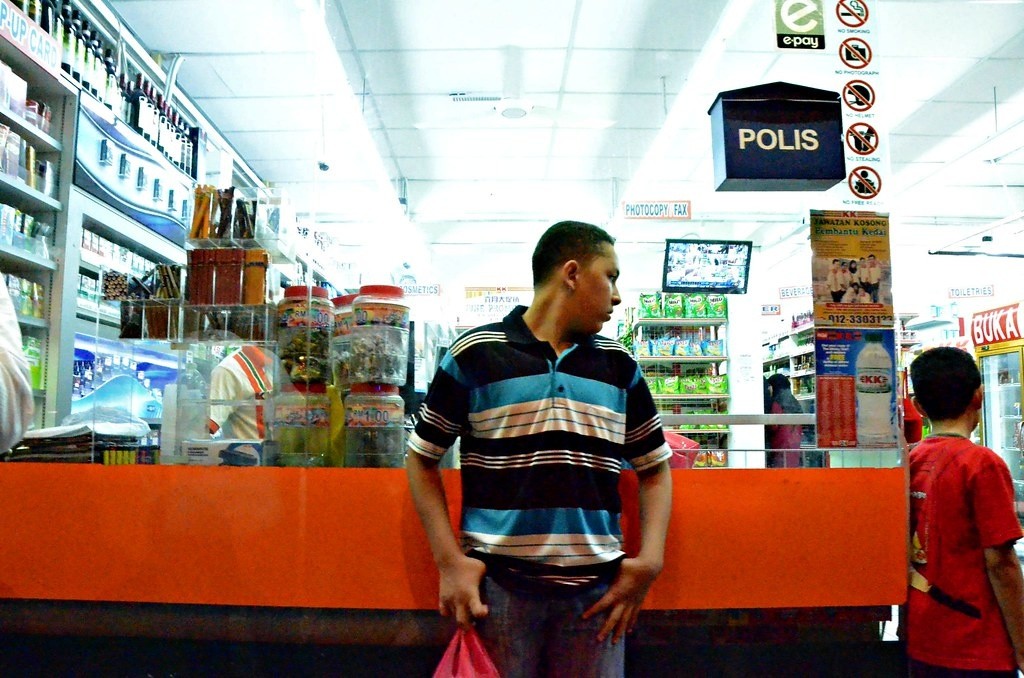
left=898, top=330, right=923, bottom=444
left=406, top=220, right=673, bottom=678
left=764, top=373, right=804, bottom=468
left=0, top=271, right=34, bottom=452
left=199, top=313, right=282, bottom=466
left=827, top=254, right=881, bottom=304
left=896, top=350, right=1024, bottom=678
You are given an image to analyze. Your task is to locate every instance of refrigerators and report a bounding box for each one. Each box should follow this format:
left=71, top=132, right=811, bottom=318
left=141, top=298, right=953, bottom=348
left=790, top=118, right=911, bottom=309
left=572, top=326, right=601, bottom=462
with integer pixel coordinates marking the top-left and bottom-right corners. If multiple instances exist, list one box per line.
left=970, top=299, right=1024, bottom=578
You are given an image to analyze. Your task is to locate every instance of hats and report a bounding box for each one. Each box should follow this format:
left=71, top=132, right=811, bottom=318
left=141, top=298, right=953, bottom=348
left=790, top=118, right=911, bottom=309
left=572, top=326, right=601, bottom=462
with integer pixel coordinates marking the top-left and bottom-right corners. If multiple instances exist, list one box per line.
left=766, top=374, right=791, bottom=399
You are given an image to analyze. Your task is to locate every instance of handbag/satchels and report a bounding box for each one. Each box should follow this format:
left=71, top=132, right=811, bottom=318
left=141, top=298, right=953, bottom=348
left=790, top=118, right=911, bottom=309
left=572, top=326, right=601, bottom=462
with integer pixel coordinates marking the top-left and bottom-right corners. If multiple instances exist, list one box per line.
left=430, top=623, right=504, bottom=678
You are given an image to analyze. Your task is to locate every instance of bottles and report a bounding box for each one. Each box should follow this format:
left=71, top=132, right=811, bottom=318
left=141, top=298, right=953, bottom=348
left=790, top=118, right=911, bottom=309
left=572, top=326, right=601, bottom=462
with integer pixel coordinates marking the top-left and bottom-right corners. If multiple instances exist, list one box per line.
left=854, top=332, right=893, bottom=446
left=10, top=0, right=193, bottom=176
left=1017, top=450, right=1024, bottom=479
left=1017, top=484, right=1024, bottom=518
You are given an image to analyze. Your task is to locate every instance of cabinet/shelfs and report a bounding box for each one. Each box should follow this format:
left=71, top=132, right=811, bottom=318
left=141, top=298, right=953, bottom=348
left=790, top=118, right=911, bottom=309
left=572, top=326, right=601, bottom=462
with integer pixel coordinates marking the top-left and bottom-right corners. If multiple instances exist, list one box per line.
left=0, top=33, right=80, bottom=432
left=268, top=251, right=310, bottom=302
left=413, top=319, right=473, bottom=467
left=617, top=285, right=1024, bottom=558
left=310, top=266, right=345, bottom=299
left=62, top=85, right=198, bottom=426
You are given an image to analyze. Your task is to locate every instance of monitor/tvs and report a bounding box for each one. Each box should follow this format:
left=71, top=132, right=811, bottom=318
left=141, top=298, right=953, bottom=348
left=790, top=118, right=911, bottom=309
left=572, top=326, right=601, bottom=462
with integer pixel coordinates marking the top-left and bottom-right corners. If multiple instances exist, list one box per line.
left=662, top=239, right=752, bottom=294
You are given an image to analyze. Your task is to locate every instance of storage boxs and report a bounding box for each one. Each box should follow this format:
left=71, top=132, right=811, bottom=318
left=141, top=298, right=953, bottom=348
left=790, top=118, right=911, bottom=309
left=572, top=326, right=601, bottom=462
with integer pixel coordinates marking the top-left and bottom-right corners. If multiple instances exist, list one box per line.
left=74, top=87, right=196, bottom=250
left=102, top=258, right=411, bottom=465
